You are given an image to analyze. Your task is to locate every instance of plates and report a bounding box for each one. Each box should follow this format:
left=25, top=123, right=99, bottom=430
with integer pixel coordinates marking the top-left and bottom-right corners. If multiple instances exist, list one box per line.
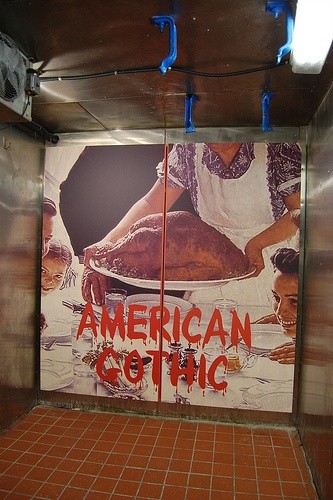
left=88, top=255, right=258, bottom=291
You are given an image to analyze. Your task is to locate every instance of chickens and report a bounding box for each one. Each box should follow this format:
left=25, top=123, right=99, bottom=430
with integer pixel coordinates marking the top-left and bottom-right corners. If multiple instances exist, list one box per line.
left=93, top=210, right=248, bottom=281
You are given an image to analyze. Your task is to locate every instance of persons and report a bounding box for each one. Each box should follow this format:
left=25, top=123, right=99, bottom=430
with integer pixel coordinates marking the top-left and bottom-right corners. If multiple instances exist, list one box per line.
left=37, top=241, right=74, bottom=297
left=249, top=246, right=298, bottom=364
left=42, top=197, right=55, bottom=253
left=83, top=143, right=301, bottom=280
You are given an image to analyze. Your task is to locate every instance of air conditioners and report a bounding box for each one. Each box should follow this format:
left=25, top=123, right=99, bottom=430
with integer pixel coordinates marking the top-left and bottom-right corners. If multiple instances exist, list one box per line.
left=0, top=32, right=34, bottom=124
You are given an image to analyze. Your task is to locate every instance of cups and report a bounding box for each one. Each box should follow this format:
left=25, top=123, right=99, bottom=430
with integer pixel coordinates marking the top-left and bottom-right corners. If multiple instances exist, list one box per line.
left=39, top=286, right=295, bottom=414
